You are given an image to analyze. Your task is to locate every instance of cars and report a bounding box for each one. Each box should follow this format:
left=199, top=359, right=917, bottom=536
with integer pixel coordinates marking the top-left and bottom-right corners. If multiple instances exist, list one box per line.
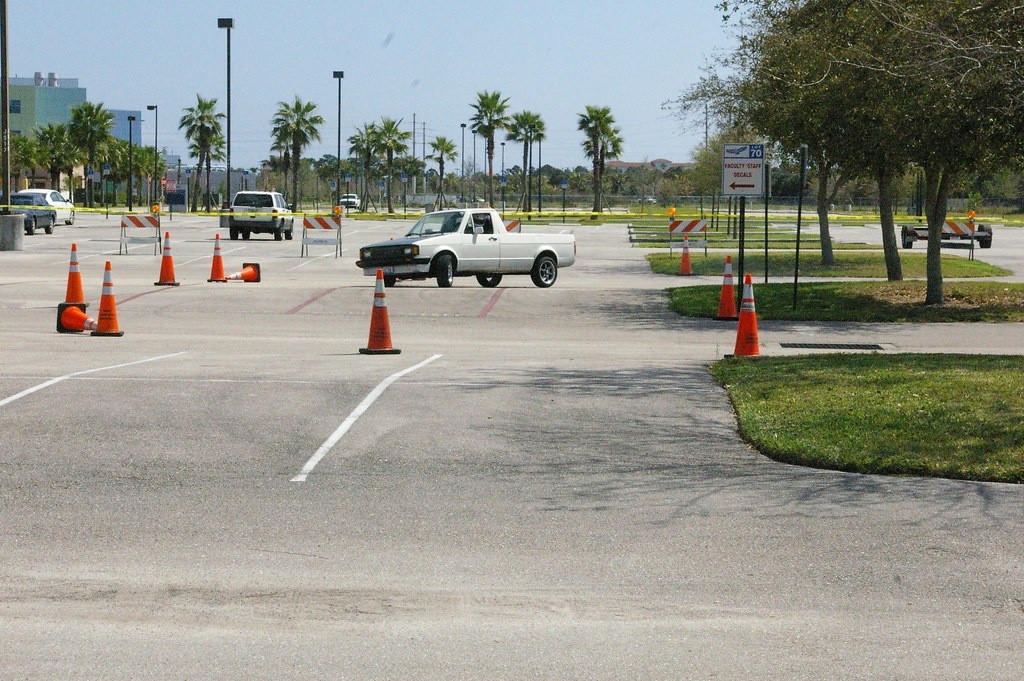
left=456, top=194, right=485, bottom=203
left=17, top=188, right=76, bottom=225
left=339, top=193, right=361, bottom=210
left=630, top=195, right=657, bottom=205
left=0, top=193, right=57, bottom=236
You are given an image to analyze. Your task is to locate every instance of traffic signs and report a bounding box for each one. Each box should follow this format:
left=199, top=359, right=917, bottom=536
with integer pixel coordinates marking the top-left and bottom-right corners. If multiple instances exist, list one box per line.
left=722, top=157, right=766, bottom=197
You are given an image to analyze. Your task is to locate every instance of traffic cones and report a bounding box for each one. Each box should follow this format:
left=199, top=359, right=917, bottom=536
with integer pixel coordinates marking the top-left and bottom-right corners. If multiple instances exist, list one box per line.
left=724, top=274, right=761, bottom=359
left=154, top=232, right=180, bottom=286
left=56, top=302, right=98, bottom=333
left=676, top=234, right=699, bottom=276
left=358, top=269, right=402, bottom=355
left=712, top=256, right=740, bottom=321
left=64, top=245, right=90, bottom=309
left=90, top=260, right=125, bottom=337
left=206, top=234, right=228, bottom=283
left=225, top=262, right=261, bottom=282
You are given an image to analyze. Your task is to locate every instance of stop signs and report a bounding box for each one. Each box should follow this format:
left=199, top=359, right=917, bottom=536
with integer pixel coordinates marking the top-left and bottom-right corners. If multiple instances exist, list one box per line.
left=161, top=176, right=168, bottom=188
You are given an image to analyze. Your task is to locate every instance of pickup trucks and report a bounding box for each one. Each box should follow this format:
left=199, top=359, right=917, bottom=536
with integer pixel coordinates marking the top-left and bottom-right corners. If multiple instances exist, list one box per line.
left=356, top=207, right=577, bottom=288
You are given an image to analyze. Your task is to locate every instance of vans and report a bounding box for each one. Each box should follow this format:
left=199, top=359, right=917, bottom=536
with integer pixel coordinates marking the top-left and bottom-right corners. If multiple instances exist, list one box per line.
left=229, top=190, right=294, bottom=240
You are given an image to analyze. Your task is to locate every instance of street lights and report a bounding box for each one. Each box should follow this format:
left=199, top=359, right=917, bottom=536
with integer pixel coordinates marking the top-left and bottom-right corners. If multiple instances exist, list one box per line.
left=472, top=130, right=478, bottom=202
left=332, top=70, right=344, bottom=215
left=483, top=134, right=489, bottom=202
left=500, top=142, right=506, bottom=212
left=460, top=123, right=467, bottom=202
left=127, top=115, right=136, bottom=211
left=147, top=104, right=158, bottom=206
left=217, top=17, right=234, bottom=208
